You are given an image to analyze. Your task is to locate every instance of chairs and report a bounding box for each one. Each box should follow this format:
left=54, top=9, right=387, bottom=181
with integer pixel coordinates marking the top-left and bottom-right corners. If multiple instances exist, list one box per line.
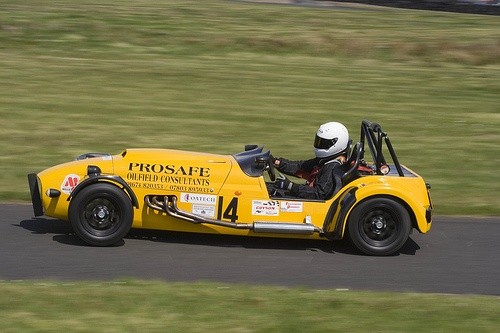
left=328, top=140, right=363, bottom=199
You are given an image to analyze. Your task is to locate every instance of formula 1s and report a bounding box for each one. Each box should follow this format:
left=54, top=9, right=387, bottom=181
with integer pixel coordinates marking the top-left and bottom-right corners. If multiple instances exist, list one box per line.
left=28, top=120, right=434, bottom=257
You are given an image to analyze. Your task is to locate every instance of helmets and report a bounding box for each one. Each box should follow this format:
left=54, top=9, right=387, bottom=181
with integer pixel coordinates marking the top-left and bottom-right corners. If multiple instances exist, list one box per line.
left=313, top=122, right=349, bottom=158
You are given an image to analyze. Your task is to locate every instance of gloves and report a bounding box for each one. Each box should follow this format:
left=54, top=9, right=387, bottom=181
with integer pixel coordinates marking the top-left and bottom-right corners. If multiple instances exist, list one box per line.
left=268, top=154, right=276, bottom=164
left=274, top=173, right=293, bottom=190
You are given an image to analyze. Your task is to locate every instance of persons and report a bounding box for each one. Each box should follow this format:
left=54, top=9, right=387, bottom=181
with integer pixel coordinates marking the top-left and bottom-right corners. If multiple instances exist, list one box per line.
left=268, top=121, right=349, bottom=200
